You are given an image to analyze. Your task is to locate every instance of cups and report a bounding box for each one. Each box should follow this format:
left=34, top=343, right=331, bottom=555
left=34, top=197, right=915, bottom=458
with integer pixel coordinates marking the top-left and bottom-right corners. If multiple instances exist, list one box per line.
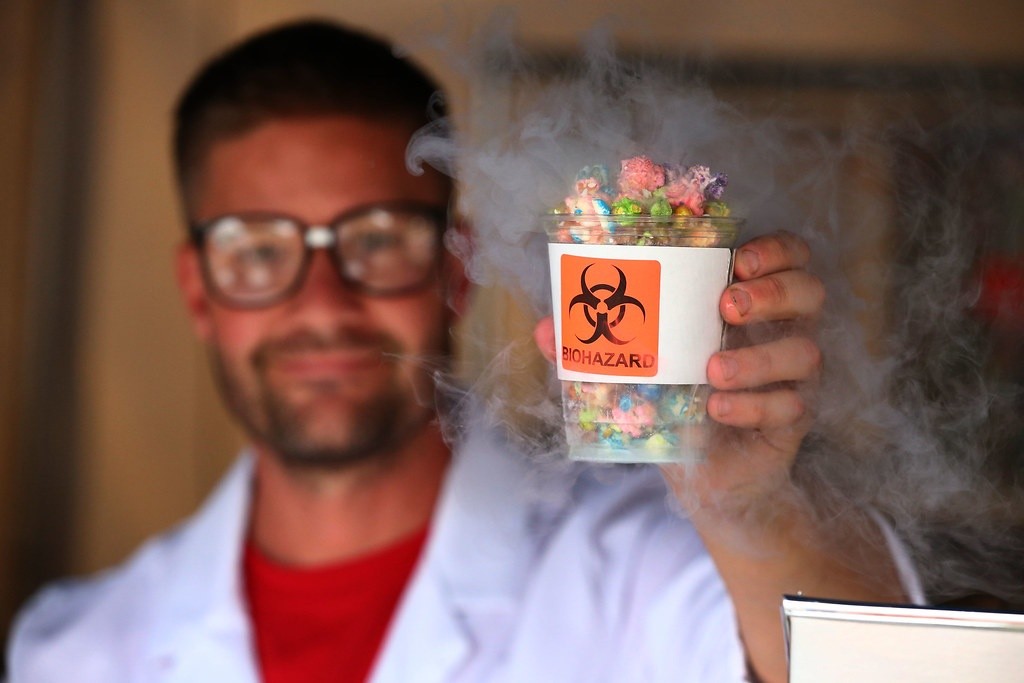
left=540, top=212, right=740, bottom=465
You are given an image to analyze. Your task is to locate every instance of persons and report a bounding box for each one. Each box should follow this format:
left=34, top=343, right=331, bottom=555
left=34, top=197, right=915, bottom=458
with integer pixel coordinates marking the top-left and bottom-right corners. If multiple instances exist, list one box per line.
left=6, top=15, right=930, bottom=683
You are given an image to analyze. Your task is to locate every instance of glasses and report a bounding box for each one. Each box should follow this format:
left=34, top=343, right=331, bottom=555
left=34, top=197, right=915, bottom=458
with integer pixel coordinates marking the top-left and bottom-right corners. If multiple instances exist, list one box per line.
left=190, top=199, right=455, bottom=311
